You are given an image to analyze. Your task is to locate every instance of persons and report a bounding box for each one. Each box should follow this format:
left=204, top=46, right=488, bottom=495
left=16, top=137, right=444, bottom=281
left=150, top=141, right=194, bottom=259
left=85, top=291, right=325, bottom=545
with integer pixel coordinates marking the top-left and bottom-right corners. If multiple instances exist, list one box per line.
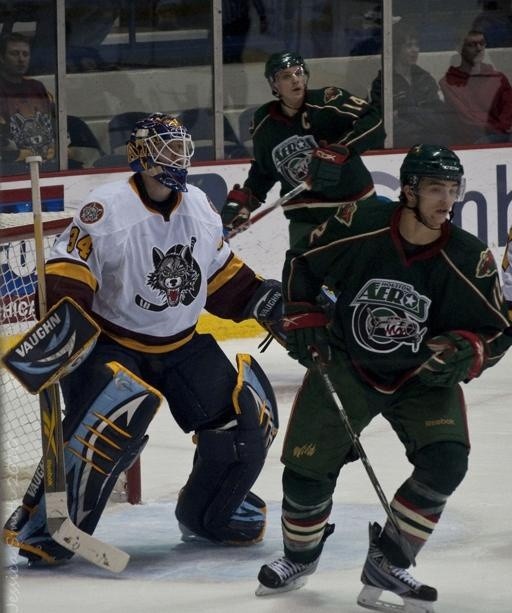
left=438, top=27, right=512, bottom=144
left=365, top=27, right=457, bottom=146
left=221, top=48, right=389, bottom=317
left=256, top=142, right=512, bottom=602
left=0, top=32, right=83, bottom=176
left=1, top=109, right=280, bottom=566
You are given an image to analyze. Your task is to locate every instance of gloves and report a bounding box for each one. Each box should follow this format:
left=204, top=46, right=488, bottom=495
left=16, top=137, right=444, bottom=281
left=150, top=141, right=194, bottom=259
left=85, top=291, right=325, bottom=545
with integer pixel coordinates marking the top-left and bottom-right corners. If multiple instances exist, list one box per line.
left=243, top=279, right=287, bottom=347
left=219, top=184, right=260, bottom=231
left=281, top=301, right=329, bottom=366
left=418, top=330, right=485, bottom=387
left=306, top=140, right=349, bottom=191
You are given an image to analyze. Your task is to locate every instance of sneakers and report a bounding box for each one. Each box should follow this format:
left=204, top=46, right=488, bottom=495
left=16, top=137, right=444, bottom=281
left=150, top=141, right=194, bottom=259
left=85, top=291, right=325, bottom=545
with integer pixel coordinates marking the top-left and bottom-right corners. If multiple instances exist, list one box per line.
left=361, top=553, right=437, bottom=601
left=257, top=546, right=336, bottom=588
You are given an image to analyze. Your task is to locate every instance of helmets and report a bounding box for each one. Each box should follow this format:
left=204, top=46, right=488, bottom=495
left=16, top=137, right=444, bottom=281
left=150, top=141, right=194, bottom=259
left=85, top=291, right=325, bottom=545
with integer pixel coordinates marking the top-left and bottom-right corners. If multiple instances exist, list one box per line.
left=400, top=142, right=464, bottom=185
left=264, top=50, right=304, bottom=82
left=127, top=113, right=194, bottom=192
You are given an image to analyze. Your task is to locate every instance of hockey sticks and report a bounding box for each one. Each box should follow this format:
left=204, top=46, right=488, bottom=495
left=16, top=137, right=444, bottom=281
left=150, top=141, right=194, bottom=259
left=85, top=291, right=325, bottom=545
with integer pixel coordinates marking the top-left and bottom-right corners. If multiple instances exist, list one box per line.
left=309, top=352, right=416, bottom=568
left=24, top=157, right=130, bottom=571
